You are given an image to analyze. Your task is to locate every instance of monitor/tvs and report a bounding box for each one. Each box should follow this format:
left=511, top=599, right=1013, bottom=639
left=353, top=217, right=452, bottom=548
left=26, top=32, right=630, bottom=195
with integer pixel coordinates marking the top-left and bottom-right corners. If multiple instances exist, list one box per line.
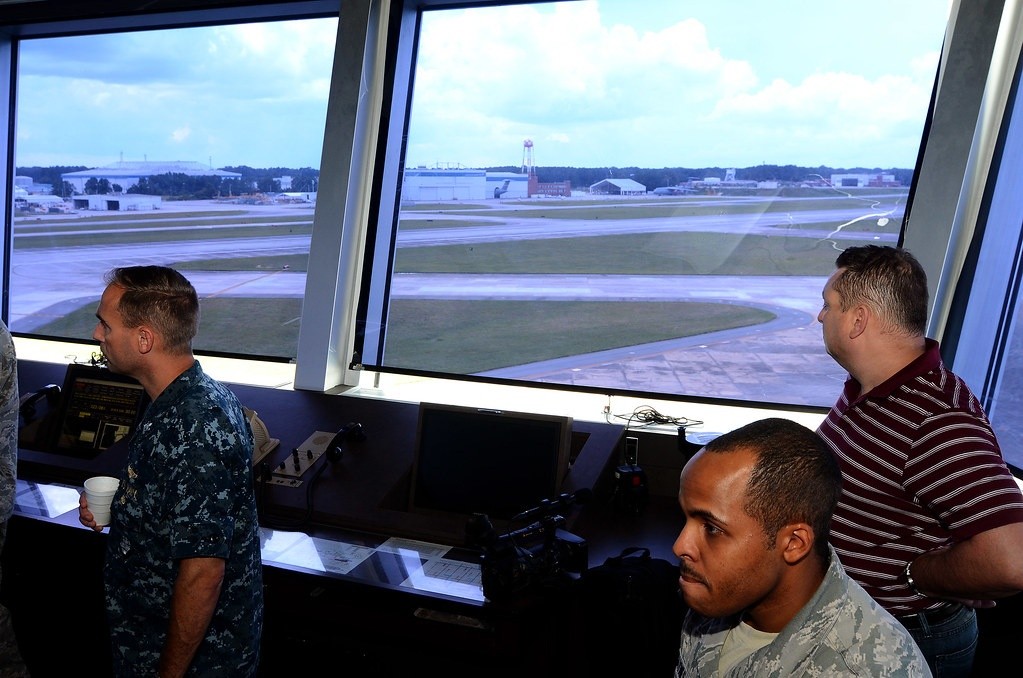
left=409, top=403, right=567, bottom=524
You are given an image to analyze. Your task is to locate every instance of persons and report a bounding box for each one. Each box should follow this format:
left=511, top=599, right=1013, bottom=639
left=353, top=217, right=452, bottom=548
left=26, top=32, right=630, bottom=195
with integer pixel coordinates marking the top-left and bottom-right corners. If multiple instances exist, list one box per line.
left=78, top=265, right=265, bottom=678
left=671, top=418, right=932, bottom=678
left=812, top=246, right=1023, bottom=678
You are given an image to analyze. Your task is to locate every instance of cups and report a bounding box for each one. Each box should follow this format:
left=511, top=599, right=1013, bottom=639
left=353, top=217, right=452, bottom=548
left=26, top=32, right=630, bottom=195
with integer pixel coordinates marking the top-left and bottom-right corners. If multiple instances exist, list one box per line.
left=83, top=476, right=120, bottom=526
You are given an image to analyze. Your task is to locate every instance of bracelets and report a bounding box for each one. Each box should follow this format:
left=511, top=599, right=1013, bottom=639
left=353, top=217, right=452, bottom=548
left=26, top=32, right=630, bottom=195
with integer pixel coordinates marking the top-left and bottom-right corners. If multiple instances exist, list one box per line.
left=903, top=562, right=927, bottom=600
